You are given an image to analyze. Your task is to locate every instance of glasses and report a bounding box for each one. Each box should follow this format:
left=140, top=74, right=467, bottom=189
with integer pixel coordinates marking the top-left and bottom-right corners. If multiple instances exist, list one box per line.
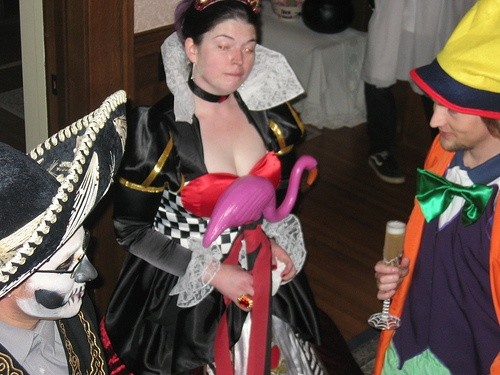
left=36, top=228, right=90, bottom=279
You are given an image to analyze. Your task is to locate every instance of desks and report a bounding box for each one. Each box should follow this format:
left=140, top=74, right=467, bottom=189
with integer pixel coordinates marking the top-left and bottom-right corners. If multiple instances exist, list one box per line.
left=260, top=0, right=369, bottom=120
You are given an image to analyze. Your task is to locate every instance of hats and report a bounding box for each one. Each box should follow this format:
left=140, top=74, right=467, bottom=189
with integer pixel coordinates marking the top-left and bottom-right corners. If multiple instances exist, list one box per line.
left=409, top=0, right=500, bottom=120
left=0, top=90, right=127, bottom=301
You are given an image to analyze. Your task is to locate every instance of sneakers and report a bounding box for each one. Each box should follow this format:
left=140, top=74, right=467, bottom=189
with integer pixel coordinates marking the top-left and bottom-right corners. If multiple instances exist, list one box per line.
left=367, top=150, right=405, bottom=184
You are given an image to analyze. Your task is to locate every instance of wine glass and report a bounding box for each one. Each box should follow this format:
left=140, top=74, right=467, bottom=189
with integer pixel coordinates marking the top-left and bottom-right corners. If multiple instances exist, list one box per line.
left=368, top=221, right=406, bottom=330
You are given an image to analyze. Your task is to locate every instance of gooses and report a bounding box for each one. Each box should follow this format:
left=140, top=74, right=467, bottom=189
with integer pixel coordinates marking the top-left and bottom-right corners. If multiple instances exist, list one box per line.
left=202, top=154, right=318, bottom=248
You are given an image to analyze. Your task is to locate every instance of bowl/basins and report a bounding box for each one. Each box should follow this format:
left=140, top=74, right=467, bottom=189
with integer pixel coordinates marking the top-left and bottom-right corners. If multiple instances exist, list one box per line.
left=272, top=0, right=302, bottom=19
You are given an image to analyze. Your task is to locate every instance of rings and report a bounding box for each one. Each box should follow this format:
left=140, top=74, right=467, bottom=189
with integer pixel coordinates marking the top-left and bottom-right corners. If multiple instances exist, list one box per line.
left=236, top=295, right=253, bottom=309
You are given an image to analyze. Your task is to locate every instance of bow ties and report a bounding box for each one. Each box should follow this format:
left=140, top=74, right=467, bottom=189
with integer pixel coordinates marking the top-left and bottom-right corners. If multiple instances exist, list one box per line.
left=416, top=168, right=493, bottom=227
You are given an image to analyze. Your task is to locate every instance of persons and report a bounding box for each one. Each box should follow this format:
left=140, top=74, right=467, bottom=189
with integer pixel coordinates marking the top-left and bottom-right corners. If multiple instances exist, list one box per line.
left=98, top=1, right=367, bottom=375
left=0, top=153, right=109, bottom=375
left=375, top=36, right=500, bottom=375
